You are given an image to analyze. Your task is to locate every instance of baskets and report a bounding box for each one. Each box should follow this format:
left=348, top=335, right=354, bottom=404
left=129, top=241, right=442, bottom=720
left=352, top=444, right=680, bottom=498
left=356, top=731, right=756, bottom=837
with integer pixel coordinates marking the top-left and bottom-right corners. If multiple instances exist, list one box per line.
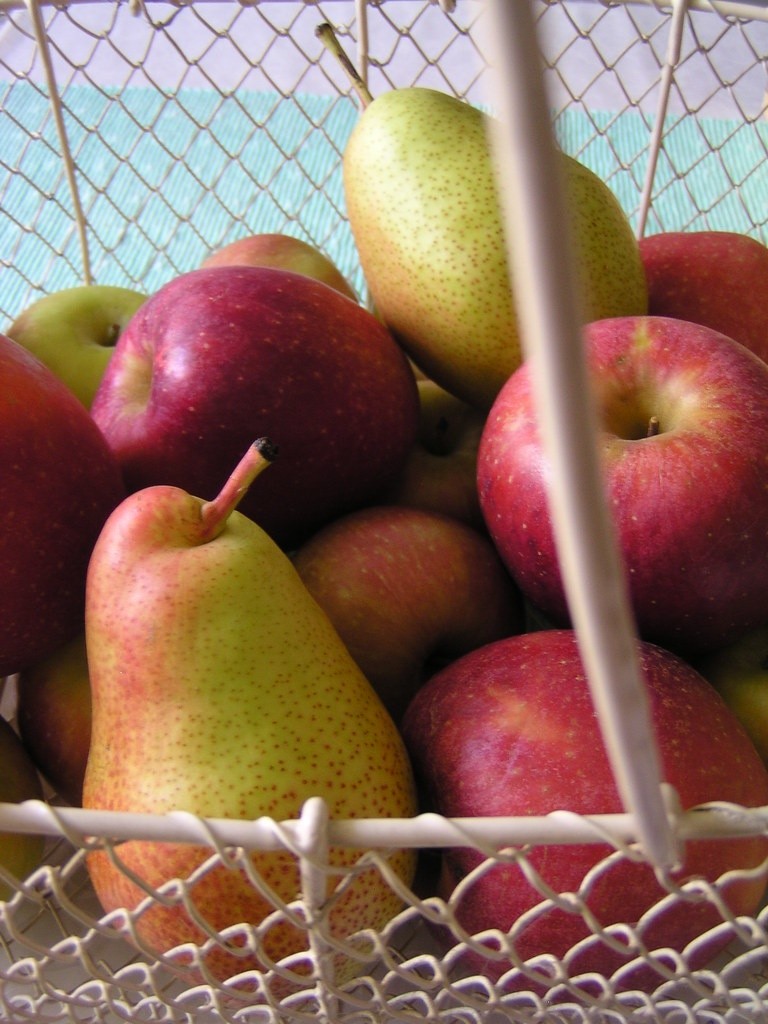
left=0, top=0, right=768, bottom=1023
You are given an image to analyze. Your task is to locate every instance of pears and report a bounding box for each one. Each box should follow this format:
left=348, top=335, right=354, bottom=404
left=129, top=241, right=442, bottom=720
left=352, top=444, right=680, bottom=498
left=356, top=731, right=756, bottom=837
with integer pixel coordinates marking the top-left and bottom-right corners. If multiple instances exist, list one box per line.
left=74, top=437, right=419, bottom=1004
left=314, top=20, right=647, bottom=408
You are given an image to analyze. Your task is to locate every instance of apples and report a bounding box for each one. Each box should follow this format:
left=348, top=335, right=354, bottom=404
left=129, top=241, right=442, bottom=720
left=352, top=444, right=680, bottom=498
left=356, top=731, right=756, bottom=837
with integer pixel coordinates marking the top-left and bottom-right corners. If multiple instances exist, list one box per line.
left=0, top=220, right=768, bottom=1008
